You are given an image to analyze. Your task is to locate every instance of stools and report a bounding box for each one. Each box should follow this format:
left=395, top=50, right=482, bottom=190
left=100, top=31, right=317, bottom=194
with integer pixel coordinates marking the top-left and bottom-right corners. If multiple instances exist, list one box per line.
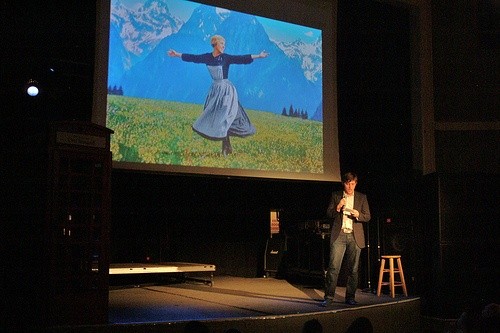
left=376, top=255, right=407, bottom=298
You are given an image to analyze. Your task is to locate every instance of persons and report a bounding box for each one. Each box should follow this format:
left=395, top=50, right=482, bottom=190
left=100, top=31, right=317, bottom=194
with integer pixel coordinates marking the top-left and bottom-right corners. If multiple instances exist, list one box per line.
left=165, top=33, right=271, bottom=153
left=322, top=169, right=371, bottom=307
left=348, top=316, right=375, bottom=333
left=478, top=303, right=500, bottom=333
left=302, top=319, right=323, bottom=333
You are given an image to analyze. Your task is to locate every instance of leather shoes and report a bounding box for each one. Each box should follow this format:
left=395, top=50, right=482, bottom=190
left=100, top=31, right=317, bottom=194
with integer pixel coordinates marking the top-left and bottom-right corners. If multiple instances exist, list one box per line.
left=322, top=298, right=334, bottom=307
left=345, top=299, right=361, bottom=307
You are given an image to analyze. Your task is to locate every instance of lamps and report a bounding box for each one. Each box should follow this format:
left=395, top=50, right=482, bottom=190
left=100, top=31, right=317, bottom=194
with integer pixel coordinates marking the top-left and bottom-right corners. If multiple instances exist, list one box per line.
left=25, top=78, right=39, bottom=97
